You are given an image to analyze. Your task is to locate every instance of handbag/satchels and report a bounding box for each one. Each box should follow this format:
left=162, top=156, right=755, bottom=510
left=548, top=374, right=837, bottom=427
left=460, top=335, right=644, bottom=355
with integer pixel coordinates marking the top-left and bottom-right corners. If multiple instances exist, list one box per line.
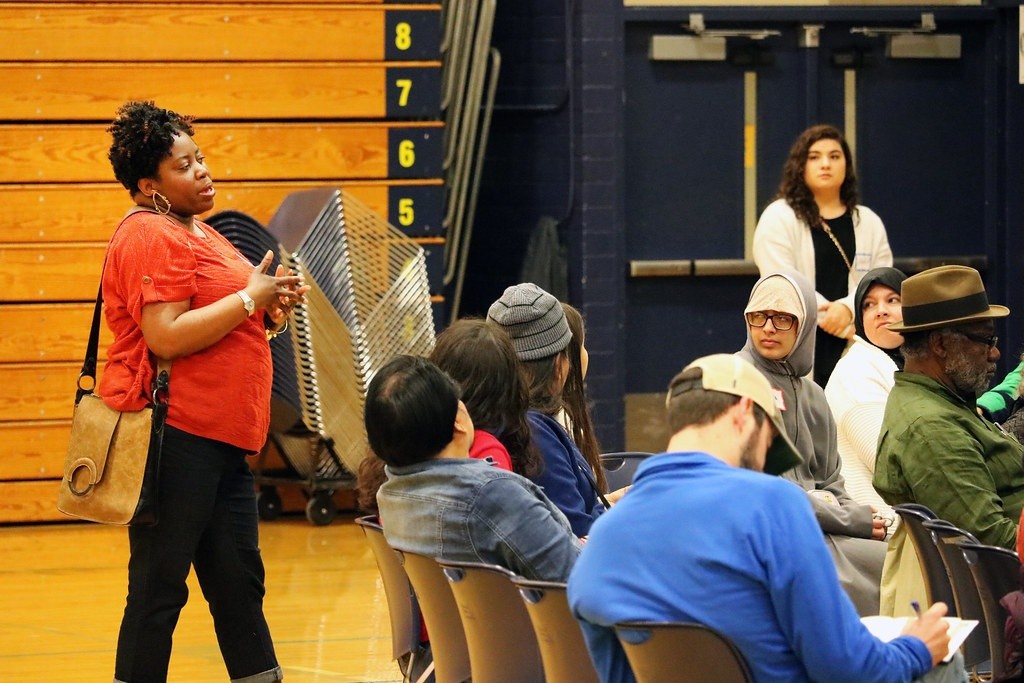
left=57, top=209, right=194, bottom=525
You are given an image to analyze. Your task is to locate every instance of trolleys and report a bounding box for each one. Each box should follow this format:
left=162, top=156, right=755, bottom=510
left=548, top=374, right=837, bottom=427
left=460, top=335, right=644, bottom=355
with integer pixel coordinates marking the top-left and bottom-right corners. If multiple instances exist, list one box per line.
left=202, top=187, right=439, bottom=526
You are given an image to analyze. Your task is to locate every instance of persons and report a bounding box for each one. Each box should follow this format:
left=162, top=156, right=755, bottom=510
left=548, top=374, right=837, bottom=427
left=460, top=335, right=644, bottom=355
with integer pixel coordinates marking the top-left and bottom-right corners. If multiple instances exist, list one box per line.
left=567, top=353, right=972, bottom=683
left=99, top=99, right=311, bottom=683
left=733, top=274, right=893, bottom=619
left=752, top=125, right=894, bottom=391
left=357, top=283, right=634, bottom=648
left=872, top=266, right=1024, bottom=567
left=824, top=268, right=909, bottom=541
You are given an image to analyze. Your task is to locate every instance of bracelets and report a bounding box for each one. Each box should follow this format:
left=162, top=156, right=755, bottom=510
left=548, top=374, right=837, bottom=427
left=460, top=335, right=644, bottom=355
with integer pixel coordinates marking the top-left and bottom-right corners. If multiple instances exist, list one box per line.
left=265, top=319, right=287, bottom=341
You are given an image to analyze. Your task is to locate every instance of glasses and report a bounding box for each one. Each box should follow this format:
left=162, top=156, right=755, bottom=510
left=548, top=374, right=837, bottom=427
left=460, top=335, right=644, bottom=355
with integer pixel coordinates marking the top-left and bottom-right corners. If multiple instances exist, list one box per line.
left=948, top=328, right=999, bottom=350
left=746, top=311, right=798, bottom=331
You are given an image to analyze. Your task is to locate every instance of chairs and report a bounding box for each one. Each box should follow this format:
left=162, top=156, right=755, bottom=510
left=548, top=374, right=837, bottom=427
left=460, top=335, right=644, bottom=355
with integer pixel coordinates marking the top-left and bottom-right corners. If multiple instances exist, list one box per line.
left=353, top=449, right=1024, bottom=683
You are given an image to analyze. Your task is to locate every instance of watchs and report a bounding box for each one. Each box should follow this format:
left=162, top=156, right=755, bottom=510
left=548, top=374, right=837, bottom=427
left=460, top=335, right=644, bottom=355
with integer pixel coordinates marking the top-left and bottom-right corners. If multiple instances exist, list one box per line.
left=236, top=290, right=254, bottom=317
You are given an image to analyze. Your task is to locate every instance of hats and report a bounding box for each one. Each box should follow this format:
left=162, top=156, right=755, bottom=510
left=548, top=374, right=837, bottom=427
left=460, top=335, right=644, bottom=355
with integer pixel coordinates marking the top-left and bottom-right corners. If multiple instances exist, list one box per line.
left=886, top=266, right=1010, bottom=331
left=664, top=353, right=805, bottom=477
left=485, top=283, right=573, bottom=360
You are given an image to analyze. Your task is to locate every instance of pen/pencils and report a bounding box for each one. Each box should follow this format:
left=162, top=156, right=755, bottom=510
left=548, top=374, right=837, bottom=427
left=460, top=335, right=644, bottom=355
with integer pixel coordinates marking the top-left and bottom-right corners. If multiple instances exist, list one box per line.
left=911, top=602, right=922, bottom=617
